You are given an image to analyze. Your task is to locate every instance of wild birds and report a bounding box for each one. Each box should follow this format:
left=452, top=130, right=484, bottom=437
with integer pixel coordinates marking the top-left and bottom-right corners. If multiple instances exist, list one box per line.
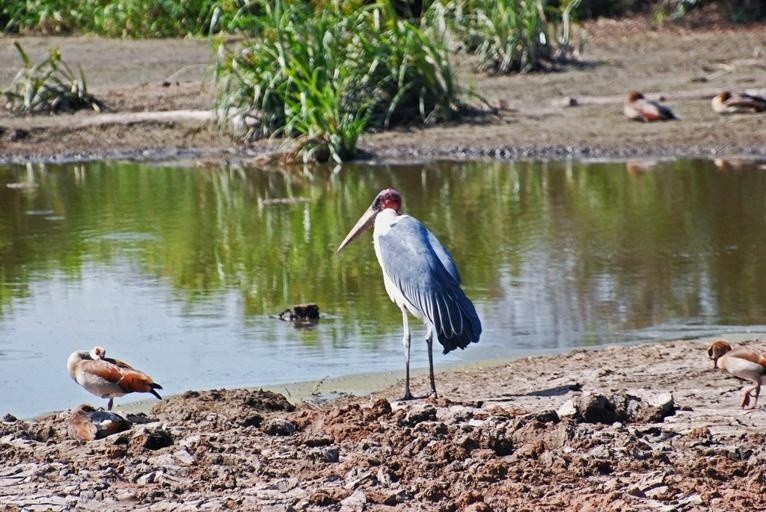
left=64, top=344, right=165, bottom=414
left=710, top=90, right=766, bottom=114
left=623, top=89, right=676, bottom=122
left=337, top=186, right=484, bottom=404
left=708, top=339, right=766, bottom=411
